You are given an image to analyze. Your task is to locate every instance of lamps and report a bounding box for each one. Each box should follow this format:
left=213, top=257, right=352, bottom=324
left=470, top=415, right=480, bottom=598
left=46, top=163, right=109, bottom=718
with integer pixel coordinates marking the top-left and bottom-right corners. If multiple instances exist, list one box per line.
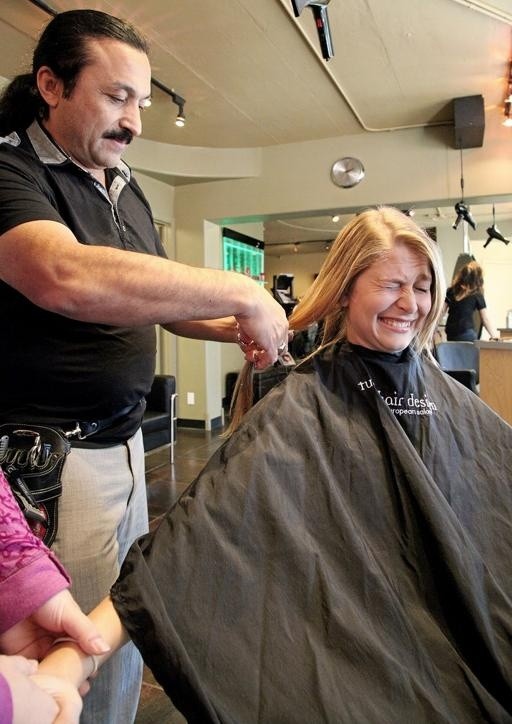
left=172, top=92, right=188, bottom=127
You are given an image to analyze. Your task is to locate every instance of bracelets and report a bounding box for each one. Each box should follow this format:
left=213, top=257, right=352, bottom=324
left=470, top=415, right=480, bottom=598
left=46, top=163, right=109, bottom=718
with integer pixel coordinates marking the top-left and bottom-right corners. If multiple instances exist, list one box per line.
left=53, top=636, right=99, bottom=674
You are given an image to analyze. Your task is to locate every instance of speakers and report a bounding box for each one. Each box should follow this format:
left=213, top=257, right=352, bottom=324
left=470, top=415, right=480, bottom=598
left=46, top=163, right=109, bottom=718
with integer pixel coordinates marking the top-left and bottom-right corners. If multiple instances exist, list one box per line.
left=453, top=94, right=485, bottom=149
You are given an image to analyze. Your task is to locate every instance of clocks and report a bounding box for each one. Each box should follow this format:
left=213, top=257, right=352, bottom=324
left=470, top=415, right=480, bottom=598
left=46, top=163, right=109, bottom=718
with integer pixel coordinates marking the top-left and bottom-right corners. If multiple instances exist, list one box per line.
left=330, top=154, right=366, bottom=188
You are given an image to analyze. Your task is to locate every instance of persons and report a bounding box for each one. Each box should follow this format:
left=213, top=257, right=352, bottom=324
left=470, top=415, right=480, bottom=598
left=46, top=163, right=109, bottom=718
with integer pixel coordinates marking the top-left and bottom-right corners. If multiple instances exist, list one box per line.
left=31, top=204, right=512, bottom=722
left=0, top=9, right=296, bottom=723
left=429, top=261, right=497, bottom=386
left=0, top=467, right=109, bottom=724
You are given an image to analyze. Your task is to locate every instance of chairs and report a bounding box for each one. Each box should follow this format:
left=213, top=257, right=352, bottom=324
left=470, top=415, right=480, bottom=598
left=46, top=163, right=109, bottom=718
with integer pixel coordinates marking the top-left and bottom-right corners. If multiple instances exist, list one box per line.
left=440, top=368, right=479, bottom=399
left=141, top=374, right=177, bottom=454
left=433, top=340, right=480, bottom=383
